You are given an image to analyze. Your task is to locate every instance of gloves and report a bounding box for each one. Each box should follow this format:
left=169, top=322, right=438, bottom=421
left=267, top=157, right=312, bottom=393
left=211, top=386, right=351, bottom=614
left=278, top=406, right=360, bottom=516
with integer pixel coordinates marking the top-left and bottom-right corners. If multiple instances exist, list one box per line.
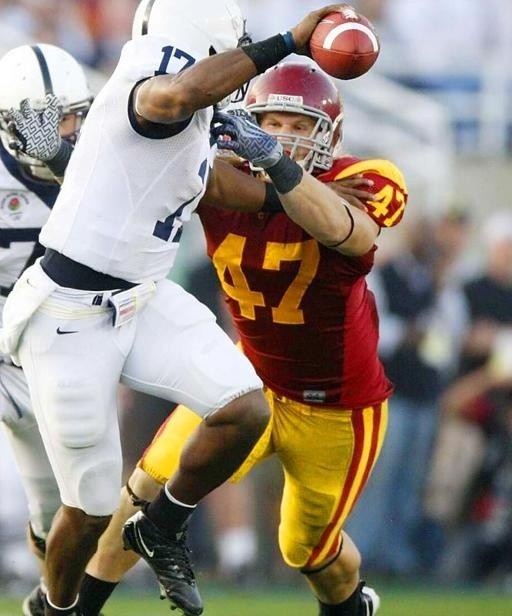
left=210, top=109, right=303, bottom=196
left=6, top=93, right=72, bottom=178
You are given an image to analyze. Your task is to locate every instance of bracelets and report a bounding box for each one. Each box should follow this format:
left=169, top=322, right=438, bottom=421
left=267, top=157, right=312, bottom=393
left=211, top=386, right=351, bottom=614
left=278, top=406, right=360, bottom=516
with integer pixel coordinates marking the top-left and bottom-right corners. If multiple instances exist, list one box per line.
left=282, top=31, right=299, bottom=54
left=242, top=33, right=290, bottom=75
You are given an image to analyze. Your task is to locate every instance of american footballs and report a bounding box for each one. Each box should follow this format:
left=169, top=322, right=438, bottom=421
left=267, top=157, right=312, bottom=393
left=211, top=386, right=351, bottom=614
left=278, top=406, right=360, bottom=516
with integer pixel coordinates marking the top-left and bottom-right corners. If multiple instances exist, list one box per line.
left=311, top=10, right=381, bottom=80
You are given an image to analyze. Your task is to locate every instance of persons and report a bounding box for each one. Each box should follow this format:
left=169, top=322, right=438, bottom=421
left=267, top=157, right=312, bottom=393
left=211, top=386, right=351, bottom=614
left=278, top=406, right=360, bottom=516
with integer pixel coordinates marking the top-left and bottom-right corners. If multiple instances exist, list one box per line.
left=82, top=63, right=381, bottom=616
left=4, top=0, right=350, bottom=616
left=346, top=195, right=511, bottom=593
left=116, top=263, right=301, bottom=594
left=5, top=44, right=98, bottom=616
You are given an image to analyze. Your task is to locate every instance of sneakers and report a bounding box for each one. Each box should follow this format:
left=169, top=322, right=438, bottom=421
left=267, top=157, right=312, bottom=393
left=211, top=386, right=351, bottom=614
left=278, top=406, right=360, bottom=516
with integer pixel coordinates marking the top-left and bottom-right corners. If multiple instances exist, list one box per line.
left=317, top=581, right=382, bottom=615
left=121, top=501, right=204, bottom=616
left=21, top=577, right=49, bottom=615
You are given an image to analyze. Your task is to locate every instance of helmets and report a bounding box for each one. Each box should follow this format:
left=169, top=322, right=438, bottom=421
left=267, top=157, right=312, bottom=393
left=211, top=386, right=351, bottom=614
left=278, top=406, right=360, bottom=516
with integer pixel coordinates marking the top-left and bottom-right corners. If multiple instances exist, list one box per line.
left=131, top=1, right=253, bottom=56
left=1, top=42, right=95, bottom=122
left=242, top=61, right=344, bottom=176
